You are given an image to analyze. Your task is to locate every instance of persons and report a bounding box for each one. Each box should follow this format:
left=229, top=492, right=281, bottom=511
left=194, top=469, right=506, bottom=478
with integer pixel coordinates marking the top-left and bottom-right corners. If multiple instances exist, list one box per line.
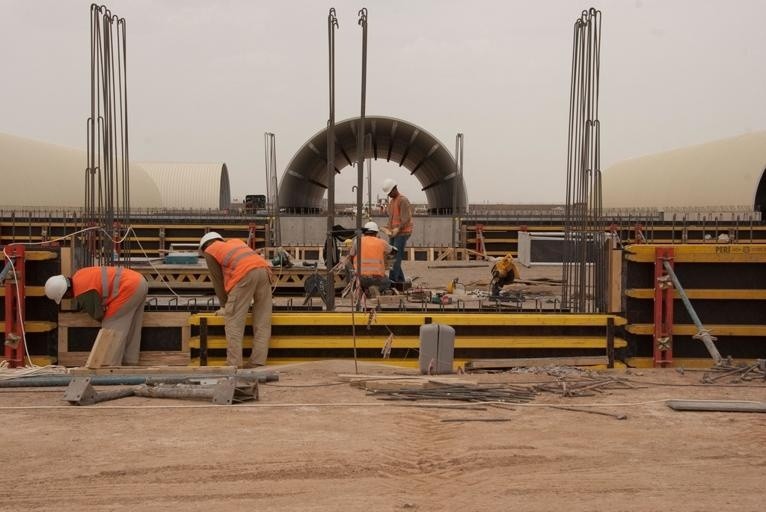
left=352, top=221, right=414, bottom=292
left=380, top=178, right=414, bottom=282
left=44, top=264, right=149, bottom=366
left=198, top=230, right=276, bottom=369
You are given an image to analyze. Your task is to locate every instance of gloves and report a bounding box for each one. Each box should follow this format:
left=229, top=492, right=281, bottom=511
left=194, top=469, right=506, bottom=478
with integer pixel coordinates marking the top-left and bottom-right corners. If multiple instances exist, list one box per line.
left=388, top=226, right=401, bottom=240
left=214, top=306, right=225, bottom=317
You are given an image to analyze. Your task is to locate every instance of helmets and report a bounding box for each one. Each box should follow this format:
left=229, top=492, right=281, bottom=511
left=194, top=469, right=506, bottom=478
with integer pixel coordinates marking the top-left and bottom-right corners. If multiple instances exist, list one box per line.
left=43, top=274, right=68, bottom=306
left=197, top=231, right=226, bottom=256
left=363, top=221, right=380, bottom=233
left=381, top=177, right=399, bottom=195
